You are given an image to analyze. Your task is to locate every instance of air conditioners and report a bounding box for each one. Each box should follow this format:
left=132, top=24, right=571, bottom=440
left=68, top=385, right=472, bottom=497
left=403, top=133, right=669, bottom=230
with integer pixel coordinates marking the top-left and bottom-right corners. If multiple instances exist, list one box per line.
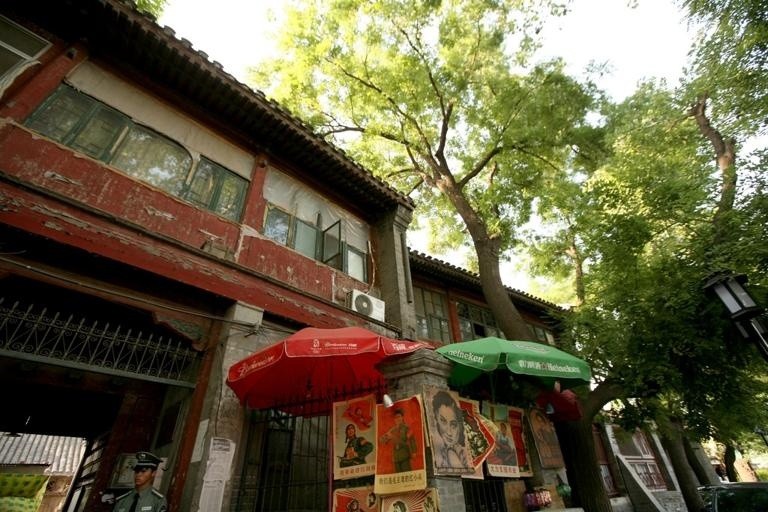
left=344, top=288, right=386, bottom=324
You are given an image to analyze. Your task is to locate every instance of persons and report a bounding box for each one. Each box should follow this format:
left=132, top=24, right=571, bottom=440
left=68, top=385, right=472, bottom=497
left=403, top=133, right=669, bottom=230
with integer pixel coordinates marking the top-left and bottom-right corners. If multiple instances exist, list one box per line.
left=535, top=412, right=559, bottom=447
left=368, top=492, right=375, bottom=506
left=379, top=409, right=416, bottom=473
left=432, top=391, right=466, bottom=468
left=392, top=501, right=407, bottom=511
left=424, top=497, right=432, bottom=509
left=340, top=425, right=372, bottom=467
left=113, top=452, right=167, bottom=511
left=495, top=422, right=512, bottom=464
left=347, top=499, right=359, bottom=511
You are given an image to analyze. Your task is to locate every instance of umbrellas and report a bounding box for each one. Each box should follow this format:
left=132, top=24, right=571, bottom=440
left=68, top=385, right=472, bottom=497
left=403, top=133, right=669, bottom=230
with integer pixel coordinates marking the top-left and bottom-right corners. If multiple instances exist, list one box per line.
left=226, top=327, right=436, bottom=418
left=434, top=336, right=592, bottom=402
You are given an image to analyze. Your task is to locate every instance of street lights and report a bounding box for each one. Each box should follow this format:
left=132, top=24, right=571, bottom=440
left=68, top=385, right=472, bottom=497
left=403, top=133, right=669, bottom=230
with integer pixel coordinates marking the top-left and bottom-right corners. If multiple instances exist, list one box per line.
left=699, top=267, right=768, bottom=368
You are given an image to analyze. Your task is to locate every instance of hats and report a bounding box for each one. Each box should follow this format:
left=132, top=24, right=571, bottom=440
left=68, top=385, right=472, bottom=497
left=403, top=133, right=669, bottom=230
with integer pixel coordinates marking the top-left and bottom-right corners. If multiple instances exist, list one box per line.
left=135, top=451, right=164, bottom=468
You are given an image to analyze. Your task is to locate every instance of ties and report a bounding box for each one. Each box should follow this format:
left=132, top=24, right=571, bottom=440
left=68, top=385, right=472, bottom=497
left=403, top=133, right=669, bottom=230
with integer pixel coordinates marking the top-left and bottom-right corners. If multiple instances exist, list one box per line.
left=129, top=492, right=140, bottom=512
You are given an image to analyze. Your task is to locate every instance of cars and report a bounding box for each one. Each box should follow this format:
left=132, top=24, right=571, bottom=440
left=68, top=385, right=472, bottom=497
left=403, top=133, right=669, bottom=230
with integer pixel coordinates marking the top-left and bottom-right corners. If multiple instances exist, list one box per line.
left=695, top=479, right=768, bottom=512
